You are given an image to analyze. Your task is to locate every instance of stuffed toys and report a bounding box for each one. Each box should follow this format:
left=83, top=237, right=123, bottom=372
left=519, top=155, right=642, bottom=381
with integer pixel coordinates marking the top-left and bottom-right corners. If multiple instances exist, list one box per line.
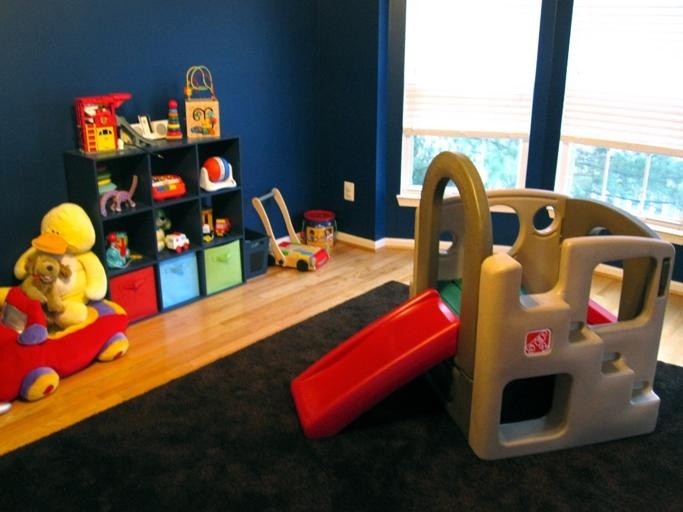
left=0, top=201, right=130, bottom=405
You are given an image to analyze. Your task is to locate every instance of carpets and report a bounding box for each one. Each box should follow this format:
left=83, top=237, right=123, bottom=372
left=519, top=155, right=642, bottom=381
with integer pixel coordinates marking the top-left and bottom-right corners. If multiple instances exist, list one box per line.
left=0, top=281, right=683, bottom=510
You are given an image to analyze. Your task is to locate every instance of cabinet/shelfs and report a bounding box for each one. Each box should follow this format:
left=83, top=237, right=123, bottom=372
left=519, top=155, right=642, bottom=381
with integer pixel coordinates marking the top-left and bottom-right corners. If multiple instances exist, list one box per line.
left=64, top=134, right=246, bottom=326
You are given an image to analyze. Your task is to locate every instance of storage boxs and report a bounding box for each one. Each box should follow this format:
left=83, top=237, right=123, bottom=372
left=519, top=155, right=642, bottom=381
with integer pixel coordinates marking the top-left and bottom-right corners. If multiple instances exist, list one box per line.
left=247, top=229, right=270, bottom=278
